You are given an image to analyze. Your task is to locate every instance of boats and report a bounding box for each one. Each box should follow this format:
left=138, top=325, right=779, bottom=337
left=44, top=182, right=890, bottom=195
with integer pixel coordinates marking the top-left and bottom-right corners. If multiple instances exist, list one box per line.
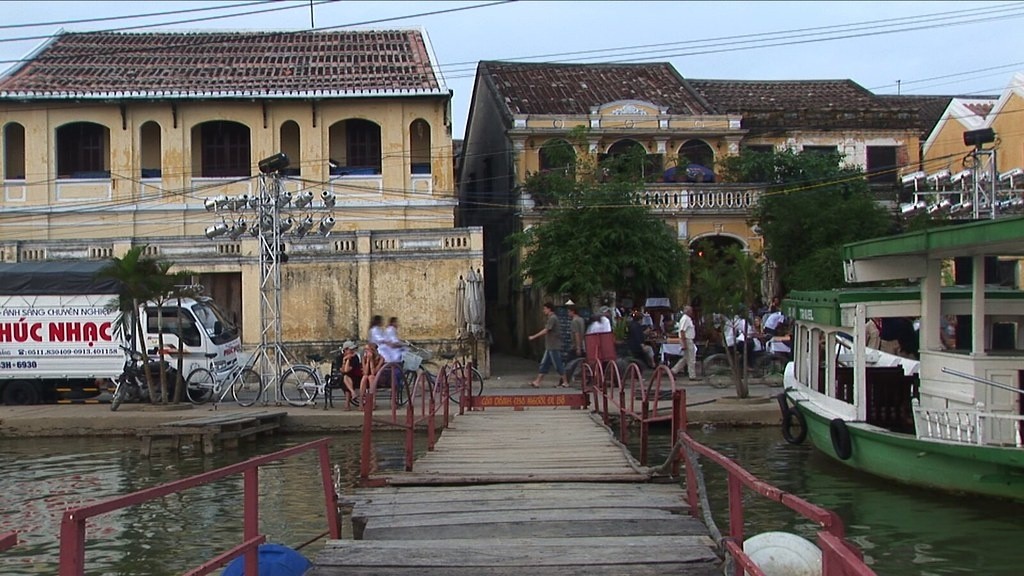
left=776, top=127, right=1024, bottom=501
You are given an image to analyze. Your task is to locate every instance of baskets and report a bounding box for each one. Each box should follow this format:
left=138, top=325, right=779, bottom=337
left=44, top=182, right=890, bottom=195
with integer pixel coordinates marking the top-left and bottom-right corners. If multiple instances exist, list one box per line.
left=401, top=352, right=423, bottom=371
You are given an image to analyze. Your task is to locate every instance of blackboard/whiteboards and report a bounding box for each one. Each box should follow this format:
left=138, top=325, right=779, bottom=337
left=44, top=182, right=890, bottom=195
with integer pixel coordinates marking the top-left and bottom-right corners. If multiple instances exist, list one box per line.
left=552, top=305, right=593, bottom=362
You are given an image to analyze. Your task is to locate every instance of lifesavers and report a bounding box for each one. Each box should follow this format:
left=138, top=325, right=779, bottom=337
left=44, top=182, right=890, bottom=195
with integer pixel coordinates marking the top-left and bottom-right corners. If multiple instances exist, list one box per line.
left=776, top=392, right=789, bottom=416
left=829, top=418, right=852, bottom=460
left=782, top=406, right=807, bottom=445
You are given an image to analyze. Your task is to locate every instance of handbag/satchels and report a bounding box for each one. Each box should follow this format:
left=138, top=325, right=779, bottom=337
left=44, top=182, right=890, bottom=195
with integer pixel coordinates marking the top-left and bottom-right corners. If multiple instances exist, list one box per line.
left=736, top=338, right=756, bottom=356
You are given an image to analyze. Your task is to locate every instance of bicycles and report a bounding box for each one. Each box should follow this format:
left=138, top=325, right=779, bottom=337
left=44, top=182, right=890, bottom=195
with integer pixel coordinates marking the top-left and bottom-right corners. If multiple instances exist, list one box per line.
left=185, top=345, right=262, bottom=408
left=279, top=346, right=345, bottom=408
left=702, top=333, right=790, bottom=389
left=394, top=339, right=483, bottom=405
left=564, top=358, right=647, bottom=390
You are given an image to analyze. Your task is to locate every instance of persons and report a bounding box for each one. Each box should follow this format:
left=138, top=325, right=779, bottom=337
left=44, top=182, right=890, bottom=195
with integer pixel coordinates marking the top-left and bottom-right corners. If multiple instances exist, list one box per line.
left=723, top=296, right=786, bottom=373
left=358, top=343, right=385, bottom=412
left=529, top=302, right=570, bottom=390
left=368, top=315, right=414, bottom=392
left=863, top=317, right=883, bottom=351
left=583, top=298, right=679, bottom=370
left=562, top=305, right=585, bottom=388
left=666, top=306, right=704, bottom=382
left=335, top=340, right=362, bottom=411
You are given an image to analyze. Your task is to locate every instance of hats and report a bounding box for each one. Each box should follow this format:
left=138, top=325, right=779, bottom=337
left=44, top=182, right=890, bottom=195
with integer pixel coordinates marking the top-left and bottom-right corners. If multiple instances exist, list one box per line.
left=343, top=341, right=358, bottom=350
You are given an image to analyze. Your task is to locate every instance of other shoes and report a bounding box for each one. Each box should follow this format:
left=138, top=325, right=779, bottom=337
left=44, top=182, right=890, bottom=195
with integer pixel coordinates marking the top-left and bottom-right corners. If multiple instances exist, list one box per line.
left=690, top=377, right=703, bottom=381
left=554, top=384, right=569, bottom=388
left=351, top=390, right=359, bottom=399
left=528, top=381, right=539, bottom=388
left=344, top=400, right=351, bottom=411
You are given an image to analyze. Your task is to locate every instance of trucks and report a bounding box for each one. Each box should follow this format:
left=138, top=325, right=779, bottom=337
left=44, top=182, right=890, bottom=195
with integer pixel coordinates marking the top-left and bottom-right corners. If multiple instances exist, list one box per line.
left=0, top=258, right=241, bottom=406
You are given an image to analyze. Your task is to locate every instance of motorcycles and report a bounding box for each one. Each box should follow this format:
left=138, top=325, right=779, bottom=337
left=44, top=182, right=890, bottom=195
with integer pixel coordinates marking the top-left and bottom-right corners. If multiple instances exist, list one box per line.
left=110, top=345, right=172, bottom=411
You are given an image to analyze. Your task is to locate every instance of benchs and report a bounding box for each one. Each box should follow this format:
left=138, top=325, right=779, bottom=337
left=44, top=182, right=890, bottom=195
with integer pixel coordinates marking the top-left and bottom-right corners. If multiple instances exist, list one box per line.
left=323, top=359, right=405, bottom=409
